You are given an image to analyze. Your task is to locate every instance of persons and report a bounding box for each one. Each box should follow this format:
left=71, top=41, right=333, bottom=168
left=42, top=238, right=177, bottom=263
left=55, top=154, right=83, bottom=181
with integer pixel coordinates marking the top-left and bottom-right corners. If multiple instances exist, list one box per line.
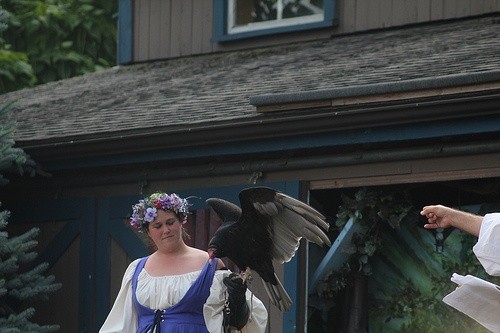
left=98, top=192, right=269, bottom=333
left=420, top=204, right=500, bottom=276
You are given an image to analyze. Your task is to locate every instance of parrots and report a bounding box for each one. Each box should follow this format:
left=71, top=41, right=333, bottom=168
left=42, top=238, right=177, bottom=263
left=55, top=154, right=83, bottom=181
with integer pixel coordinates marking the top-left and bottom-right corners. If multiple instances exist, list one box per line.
left=200, top=185, right=333, bottom=308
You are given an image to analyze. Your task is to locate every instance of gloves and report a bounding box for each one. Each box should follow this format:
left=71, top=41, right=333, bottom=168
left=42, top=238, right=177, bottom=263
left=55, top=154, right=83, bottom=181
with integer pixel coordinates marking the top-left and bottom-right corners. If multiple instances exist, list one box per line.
left=223, top=273, right=250, bottom=330
